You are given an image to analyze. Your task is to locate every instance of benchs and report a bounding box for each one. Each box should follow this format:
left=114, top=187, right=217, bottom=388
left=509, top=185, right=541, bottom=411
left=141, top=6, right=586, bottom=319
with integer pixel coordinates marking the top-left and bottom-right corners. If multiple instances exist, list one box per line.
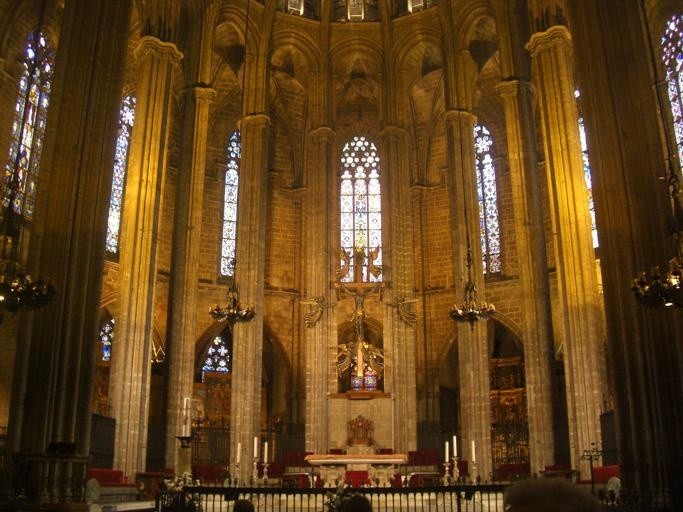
left=190, top=464, right=225, bottom=484
left=489, top=462, right=529, bottom=482
left=539, top=464, right=571, bottom=479
left=574, top=464, right=620, bottom=496
left=86, top=470, right=141, bottom=503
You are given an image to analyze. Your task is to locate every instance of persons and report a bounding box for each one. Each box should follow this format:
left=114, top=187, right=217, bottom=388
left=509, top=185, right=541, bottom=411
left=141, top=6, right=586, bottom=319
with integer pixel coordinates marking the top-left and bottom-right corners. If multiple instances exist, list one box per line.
left=386, top=296, right=419, bottom=327
left=233, top=499, right=254, bottom=512
left=344, top=496, right=370, bottom=512
left=505, top=479, right=603, bottom=511
left=338, top=282, right=381, bottom=342
left=300, top=296, right=335, bottom=328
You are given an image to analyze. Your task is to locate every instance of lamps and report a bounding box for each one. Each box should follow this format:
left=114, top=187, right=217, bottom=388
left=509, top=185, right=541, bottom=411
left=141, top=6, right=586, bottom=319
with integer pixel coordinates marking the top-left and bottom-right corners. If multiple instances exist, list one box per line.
left=446, top=0, right=497, bottom=326
left=0, top=1, right=57, bottom=316
left=207, top=1, right=258, bottom=324
left=630, top=0, right=683, bottom=310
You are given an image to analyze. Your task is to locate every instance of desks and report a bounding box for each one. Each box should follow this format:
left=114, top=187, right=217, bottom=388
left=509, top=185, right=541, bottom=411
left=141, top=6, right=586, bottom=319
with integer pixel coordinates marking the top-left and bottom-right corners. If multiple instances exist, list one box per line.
left=305, top=453, right=408, bottom=470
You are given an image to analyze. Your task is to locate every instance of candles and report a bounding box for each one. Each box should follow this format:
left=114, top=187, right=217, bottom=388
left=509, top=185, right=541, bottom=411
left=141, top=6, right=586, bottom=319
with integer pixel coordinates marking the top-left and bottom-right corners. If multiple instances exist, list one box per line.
left=254, top=436, right=258, bottom=458
left=471, top=440, right=476, bottom=462
left=264, top=442, right=268, bottom=462
left=445, top=441, right=449, bottom=462
left=237, top=442, right=241, bottom=463
left=453, top=435, right=457, bottom=457
